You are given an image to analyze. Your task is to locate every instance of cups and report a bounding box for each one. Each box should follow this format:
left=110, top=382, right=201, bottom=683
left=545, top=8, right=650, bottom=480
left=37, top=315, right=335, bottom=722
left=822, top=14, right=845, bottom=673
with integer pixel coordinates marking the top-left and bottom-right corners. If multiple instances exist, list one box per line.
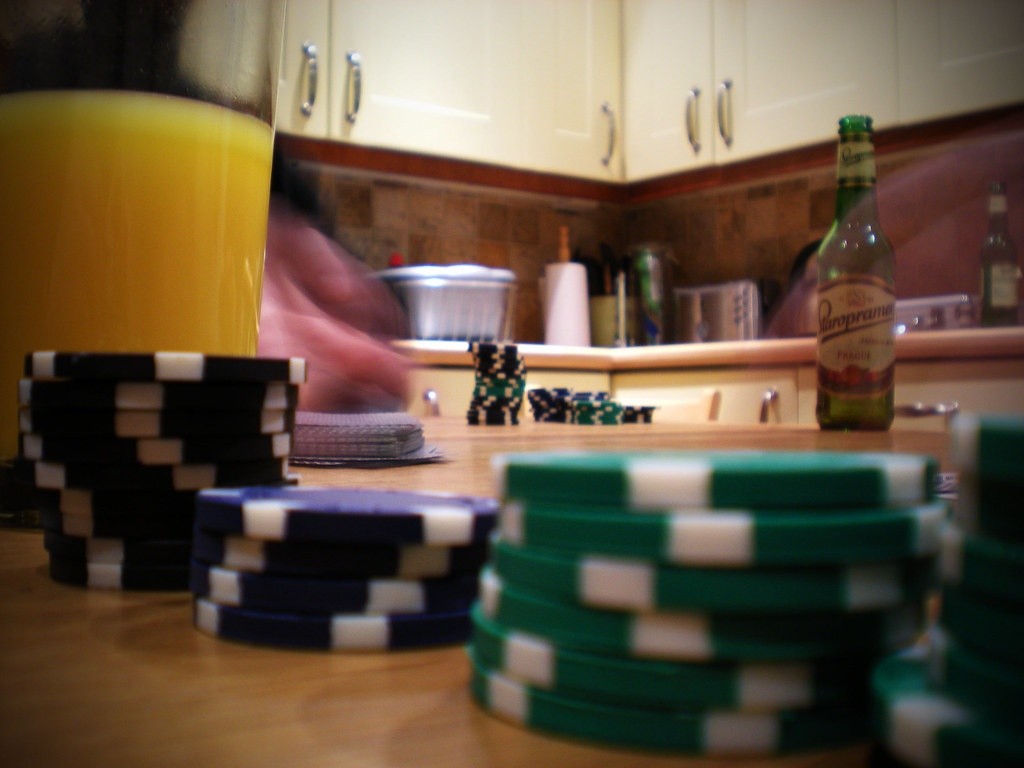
left=0, top=0, right=285, bottom=529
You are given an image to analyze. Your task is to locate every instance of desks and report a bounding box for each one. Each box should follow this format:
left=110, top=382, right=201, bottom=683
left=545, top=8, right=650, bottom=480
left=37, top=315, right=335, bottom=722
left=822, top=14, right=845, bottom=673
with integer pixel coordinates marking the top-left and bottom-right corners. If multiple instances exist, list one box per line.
left=0, top=425, right=976, bottom=768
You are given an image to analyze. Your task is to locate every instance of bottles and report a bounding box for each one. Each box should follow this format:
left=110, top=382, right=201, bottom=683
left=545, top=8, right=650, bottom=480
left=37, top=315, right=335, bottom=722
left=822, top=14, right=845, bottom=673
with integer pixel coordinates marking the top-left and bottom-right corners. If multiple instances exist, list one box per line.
left=971, top=182, right=1023, bottom=325
left=816, top=116, right=895, bottom=434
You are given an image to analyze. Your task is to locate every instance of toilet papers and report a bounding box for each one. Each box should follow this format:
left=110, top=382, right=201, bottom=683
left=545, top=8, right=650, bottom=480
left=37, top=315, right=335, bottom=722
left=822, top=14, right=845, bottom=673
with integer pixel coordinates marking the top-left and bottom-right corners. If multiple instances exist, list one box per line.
left=545, top=262, right=591, bottom=347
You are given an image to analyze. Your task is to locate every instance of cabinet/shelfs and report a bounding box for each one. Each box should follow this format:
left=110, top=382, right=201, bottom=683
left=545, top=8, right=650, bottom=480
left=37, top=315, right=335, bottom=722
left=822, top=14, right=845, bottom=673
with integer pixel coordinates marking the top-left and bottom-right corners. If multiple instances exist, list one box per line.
left=374, top=337, right=613, bottom=424
left=180, top=0, right=621, bottom=198
left=611, top=327, right=1024, bottom=432
left=623, top=0, right=1024, bottom=199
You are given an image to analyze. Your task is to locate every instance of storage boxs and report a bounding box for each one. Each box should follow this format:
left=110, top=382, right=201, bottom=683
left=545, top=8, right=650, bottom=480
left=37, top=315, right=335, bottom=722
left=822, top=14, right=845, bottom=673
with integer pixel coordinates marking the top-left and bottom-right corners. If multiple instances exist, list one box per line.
left=374, top=263, right=516, bottom=339
